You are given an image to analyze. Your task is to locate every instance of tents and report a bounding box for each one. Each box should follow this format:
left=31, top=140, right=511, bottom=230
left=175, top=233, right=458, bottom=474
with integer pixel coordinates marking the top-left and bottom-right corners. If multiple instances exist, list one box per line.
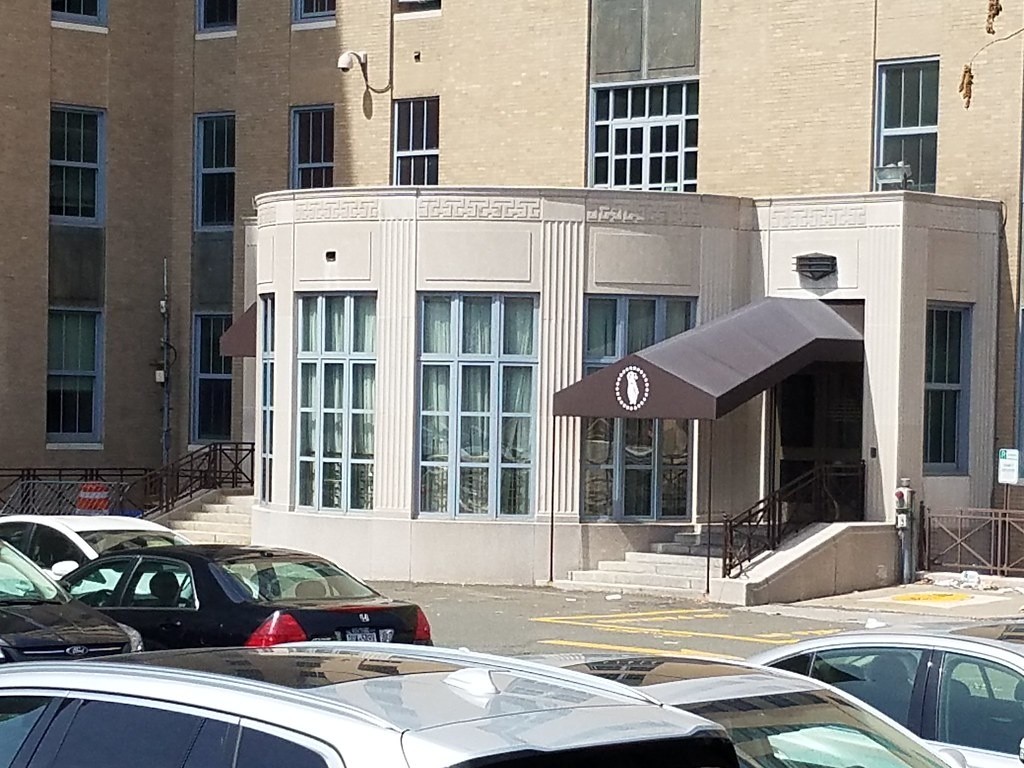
left=549, top=296, right=866, bottom=596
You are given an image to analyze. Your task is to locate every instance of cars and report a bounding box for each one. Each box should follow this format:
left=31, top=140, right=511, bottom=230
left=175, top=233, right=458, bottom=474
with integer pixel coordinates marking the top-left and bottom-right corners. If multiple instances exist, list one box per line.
left=508, top=653, right=967, bottom=768
left=745, top=632, right=1024, bottom=768
left=0, top=642, right=740, bottom=767
left=59, top=544, right=434, bottom=651
left=0, top=539, right=145, bottom=664
left=2, top=514, right=195, bottom=580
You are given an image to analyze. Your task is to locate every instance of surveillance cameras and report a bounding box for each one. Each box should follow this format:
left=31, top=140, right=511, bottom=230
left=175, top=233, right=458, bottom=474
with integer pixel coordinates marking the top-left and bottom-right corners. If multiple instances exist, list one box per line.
left=337, top=51, right=366, bottom=72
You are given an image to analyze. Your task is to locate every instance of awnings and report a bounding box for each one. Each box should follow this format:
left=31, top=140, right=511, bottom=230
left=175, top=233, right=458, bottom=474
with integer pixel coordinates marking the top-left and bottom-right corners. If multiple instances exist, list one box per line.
left=218, top=302, right=256, bottom=357
